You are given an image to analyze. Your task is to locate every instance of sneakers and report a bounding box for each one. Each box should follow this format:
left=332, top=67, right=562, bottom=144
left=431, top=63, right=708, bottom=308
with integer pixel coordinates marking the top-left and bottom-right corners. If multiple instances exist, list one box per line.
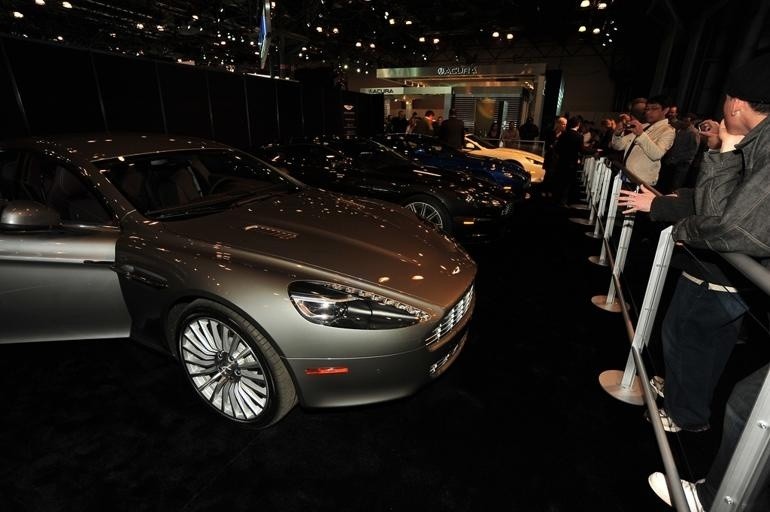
left=644, top=407, right=683, bottom=433
left=649, top=375, right=665, bottom=399
left=648, top=472, right=706, bottom=512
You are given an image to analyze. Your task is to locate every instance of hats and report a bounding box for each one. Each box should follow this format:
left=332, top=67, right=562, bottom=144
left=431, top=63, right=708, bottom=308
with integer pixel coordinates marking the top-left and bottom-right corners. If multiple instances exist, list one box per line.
left=721, top=52, right=770, bottom=105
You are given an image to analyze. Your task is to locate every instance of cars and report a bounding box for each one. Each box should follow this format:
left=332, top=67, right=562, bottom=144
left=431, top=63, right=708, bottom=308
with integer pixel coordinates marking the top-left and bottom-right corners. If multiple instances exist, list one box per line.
left=2, top=125, right=480, bottom=435
left=458, top=133, right=547, bottom=186
left=256, top=137, right=519, bottom=246
left=377, top=132, right=532, bottom=206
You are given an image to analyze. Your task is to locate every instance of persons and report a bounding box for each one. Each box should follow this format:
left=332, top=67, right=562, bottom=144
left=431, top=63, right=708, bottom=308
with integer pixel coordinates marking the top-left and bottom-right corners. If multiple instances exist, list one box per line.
left=486, top=118, right=539, bottom=148
left=383, top=108, right=464, bottom=147
left=547, top=53, right=770, bottom=512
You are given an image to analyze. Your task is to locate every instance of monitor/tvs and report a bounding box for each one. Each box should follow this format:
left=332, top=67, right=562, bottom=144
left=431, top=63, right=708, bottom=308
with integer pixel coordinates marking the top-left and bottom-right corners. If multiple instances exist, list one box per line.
left=253, top=1, right=276, bottom=71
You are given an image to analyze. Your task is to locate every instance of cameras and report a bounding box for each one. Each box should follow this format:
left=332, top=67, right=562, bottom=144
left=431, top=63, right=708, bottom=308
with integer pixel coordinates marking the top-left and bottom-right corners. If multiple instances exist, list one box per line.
left=622, top=120, right=636, bottom=132
